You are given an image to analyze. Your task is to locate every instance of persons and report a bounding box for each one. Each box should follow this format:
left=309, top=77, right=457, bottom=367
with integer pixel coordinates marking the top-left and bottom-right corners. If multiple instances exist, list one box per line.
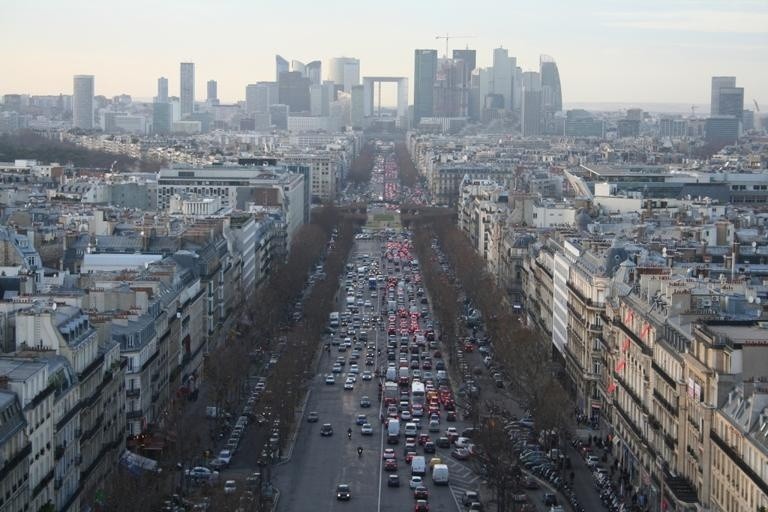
left=185, top=378, right=199, bottom=402
left=347, top=427, right=352, bottom=436
left=357, top=446, right=363, bottom=453
